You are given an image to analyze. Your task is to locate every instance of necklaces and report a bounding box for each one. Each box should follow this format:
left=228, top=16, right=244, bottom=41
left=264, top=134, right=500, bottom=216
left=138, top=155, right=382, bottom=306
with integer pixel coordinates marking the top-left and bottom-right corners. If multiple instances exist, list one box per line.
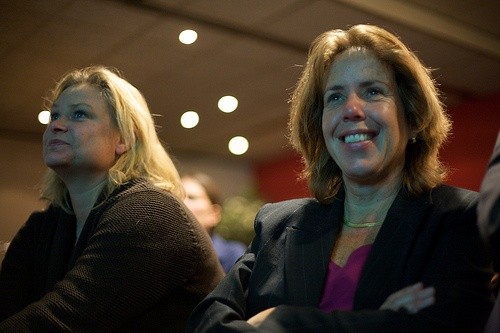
left=341, top=219, right=383, bottom=228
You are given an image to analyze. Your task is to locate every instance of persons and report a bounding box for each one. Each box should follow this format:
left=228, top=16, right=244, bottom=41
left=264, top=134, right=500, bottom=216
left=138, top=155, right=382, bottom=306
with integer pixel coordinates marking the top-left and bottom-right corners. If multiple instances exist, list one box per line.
left=0, top=66, right=226, bottom=333
left=174, top=173, right=246, bottom=279
left=187, top=22, right=500, bottom=333
